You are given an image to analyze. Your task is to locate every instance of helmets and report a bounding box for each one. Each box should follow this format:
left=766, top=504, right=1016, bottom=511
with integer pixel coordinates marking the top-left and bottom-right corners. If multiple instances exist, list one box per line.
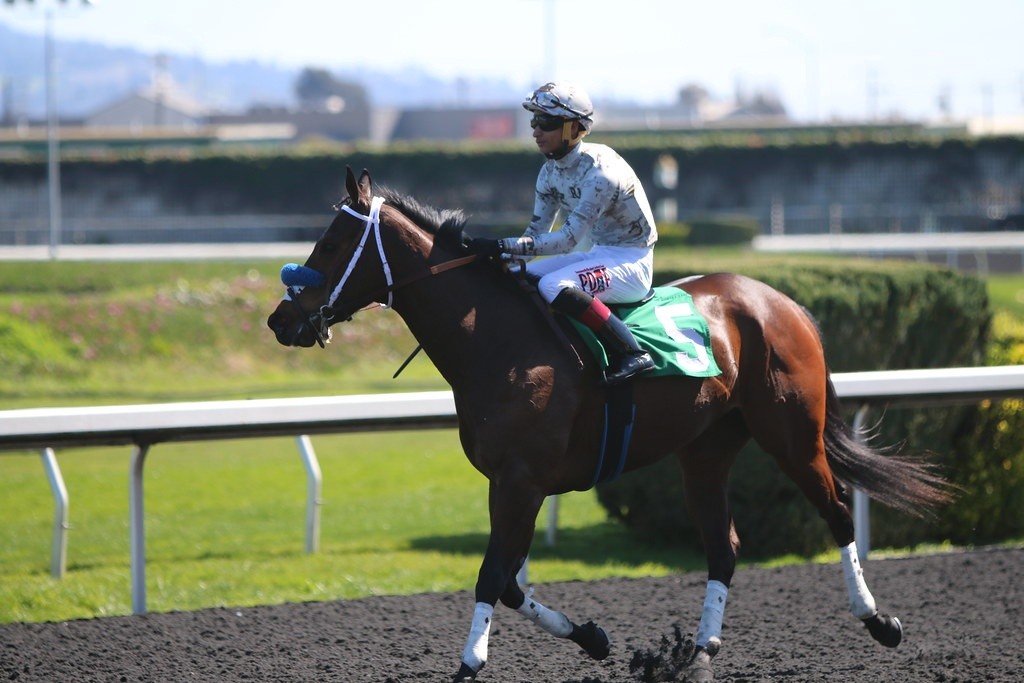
left=522, top=82, right=593, bottom=135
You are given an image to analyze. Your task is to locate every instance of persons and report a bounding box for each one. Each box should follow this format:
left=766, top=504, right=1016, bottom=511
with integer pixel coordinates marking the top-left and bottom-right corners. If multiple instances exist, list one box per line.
left=465, top=81, right=658, bottom=384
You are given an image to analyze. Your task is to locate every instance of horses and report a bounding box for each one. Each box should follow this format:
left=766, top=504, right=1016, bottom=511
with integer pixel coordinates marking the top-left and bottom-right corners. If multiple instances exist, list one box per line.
left=267, top=161, right=971, bottom=683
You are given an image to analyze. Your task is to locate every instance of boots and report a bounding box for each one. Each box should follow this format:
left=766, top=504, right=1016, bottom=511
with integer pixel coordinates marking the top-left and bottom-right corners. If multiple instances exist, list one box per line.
left=600, top=313, right=655, bottom=386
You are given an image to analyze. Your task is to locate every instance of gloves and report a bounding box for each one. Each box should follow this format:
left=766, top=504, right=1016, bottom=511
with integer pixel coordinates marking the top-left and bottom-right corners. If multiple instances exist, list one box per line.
left=468, top=237, right=504, bottom=257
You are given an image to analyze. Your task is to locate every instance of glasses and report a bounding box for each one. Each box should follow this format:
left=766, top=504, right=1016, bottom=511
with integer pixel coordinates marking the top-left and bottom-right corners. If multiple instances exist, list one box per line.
left=531, top=114, right=579, bottom=132
